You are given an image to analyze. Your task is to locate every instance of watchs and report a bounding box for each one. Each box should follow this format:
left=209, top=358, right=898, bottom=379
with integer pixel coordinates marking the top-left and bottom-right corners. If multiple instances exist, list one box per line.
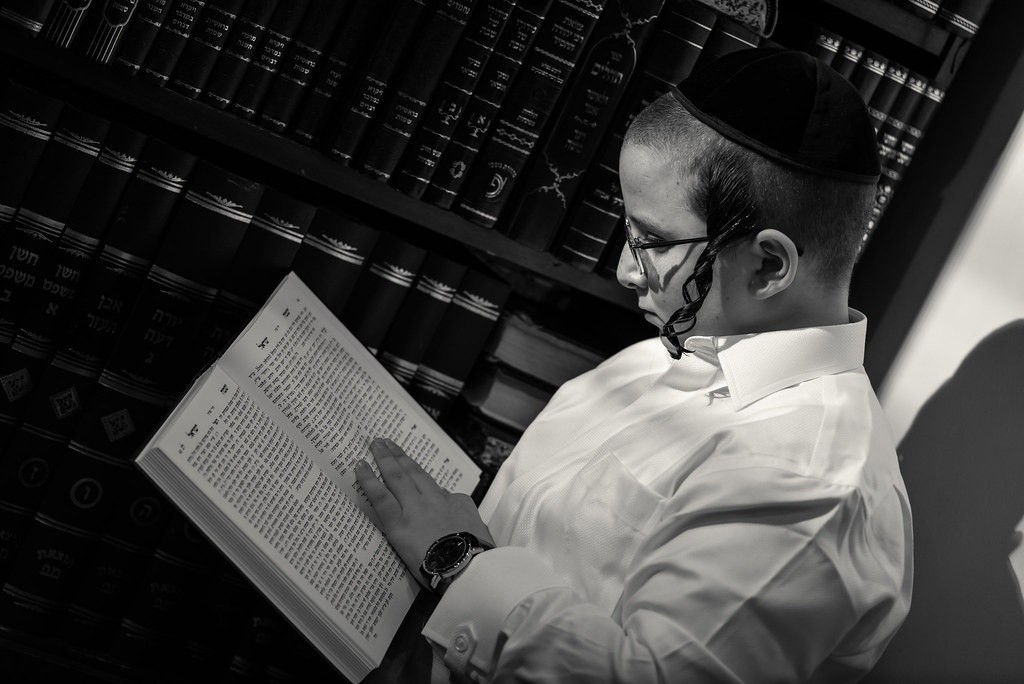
left=419, top=531, right=494, bottom=597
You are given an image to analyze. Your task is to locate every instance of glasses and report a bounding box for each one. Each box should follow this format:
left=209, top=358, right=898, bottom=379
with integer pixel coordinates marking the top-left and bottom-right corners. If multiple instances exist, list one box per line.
left=620, top=208, right=803, bottom=275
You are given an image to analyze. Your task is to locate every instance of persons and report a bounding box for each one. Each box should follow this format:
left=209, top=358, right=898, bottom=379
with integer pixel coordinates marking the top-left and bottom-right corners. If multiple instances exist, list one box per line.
left=355, top=46, right=914, bottom=684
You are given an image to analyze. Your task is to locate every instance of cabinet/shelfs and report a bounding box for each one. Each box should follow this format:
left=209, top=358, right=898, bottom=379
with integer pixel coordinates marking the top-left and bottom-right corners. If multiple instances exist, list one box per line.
left=1, top=0, right=1023, bottom=684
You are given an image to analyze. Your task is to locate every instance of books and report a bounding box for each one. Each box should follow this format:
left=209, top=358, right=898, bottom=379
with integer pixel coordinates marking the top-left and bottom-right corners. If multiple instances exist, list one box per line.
left=0, top=0, right=993, bottom=683
left=135, top=271, right=484, bottom=684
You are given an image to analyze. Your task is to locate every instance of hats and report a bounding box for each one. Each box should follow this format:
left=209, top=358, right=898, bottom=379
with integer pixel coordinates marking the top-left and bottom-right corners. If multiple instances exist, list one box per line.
left=673, top=46, right=882, bottom=184
